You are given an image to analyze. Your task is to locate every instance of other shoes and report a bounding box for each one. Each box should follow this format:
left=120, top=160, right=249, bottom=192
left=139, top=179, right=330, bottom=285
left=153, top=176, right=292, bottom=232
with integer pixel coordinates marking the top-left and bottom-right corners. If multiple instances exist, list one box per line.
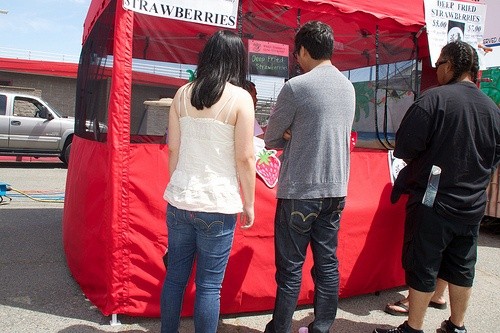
left=298, top=326, right=309, bottom=333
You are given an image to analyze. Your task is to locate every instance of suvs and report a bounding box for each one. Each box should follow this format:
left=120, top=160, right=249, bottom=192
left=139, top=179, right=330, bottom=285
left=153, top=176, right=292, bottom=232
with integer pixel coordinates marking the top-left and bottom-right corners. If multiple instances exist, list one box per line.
left=0, top=91, right=108, bottom=166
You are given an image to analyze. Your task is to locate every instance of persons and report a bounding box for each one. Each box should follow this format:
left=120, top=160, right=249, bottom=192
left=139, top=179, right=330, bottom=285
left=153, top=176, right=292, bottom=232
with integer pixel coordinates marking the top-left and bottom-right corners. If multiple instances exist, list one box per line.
left=372, top=42, right=500, bottom=333
left=263, top=20, right=356, bottom=333
left=157, top=30, right=256, bottom=333
left=231, top=79, right=267, bottom=139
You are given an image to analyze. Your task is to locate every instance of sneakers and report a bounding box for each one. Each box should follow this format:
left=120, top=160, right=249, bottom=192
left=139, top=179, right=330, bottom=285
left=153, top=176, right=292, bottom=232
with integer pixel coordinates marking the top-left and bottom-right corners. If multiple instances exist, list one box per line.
left=435, top=320, right=468, bottom=333
left=372, top=320, right=424, bottom=333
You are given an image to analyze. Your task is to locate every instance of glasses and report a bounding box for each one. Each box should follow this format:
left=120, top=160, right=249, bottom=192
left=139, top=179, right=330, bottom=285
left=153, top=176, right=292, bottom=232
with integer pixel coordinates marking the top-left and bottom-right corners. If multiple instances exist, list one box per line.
left=293, top=49, right=300, bottom=59
left=435, top=60, right=447, bottom=70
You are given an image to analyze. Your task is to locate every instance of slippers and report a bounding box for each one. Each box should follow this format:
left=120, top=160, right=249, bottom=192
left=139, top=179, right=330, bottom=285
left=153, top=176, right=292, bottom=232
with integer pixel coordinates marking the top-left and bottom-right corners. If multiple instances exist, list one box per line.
left=384, top=301, right=409, bottom=316
left=429, top=300, right=447, bottom=309
